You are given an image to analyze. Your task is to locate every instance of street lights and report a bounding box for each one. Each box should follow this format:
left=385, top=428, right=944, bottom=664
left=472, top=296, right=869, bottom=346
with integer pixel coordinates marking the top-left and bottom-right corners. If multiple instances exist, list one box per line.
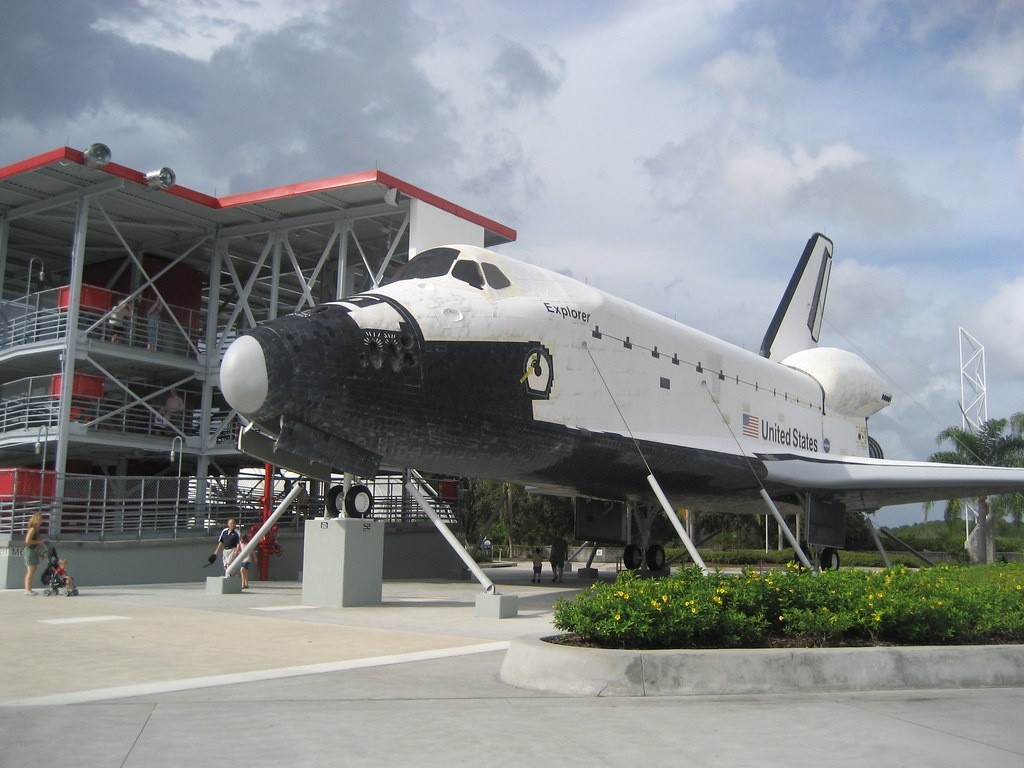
left=170, top=435, right=183, bottom=539
left=23, top=256, right=45, bottom=343
left=30, top=424, right=48, bottom=515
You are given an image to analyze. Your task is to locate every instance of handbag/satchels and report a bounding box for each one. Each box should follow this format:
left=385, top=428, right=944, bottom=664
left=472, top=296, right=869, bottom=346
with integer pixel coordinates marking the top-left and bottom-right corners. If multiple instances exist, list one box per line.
left=208, top=554, right=217, bottom=563
left=41, top=568, right=50, bottom=585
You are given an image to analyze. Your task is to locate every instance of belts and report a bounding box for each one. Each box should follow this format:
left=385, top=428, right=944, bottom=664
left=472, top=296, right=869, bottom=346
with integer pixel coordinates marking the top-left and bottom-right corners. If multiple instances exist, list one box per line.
left=224, top=546, right=232, bottom=549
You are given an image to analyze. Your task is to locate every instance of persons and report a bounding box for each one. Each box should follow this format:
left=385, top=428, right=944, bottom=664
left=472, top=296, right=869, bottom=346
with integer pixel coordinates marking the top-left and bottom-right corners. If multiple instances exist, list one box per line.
left=110, top=297, right=187, bottom=437
left=284, top=481, right=310, bottom=521
left=214, top=519, right=258, bottom=589
left=25, top=513, right=48, bottom=595
left=55, top=559, right=74, bottom=588
left=532, top=548, right=543, bottom=583
left=548, top=531, right=568, bottom=583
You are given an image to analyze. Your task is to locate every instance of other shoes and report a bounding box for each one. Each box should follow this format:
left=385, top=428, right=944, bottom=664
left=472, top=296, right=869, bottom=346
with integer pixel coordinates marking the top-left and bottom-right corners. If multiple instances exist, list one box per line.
left=552, top=576, right=558, bottom=582
left=557, top=580, right=562, bottom=584
left=24, top=591, right=36, bottom=596
left=538, top=579, right=541, bottom=583
left=242, top=585, right=248, bottom=589
left=531, top=579, right=535, bottom=583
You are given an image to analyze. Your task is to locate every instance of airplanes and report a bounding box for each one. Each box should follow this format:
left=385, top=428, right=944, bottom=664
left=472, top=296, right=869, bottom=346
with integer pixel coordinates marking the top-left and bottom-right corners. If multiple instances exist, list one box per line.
left=219, top=233, right=1024, bottom=572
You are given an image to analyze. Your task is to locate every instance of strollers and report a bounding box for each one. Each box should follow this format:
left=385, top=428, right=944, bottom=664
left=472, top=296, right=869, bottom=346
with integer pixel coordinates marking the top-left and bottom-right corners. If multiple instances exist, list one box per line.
left=34, top=541, right=78, bottom=597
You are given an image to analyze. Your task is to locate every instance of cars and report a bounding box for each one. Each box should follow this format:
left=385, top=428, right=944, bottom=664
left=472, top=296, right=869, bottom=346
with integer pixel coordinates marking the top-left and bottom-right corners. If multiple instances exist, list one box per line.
left=191, top=421, right=235, bottom=444
